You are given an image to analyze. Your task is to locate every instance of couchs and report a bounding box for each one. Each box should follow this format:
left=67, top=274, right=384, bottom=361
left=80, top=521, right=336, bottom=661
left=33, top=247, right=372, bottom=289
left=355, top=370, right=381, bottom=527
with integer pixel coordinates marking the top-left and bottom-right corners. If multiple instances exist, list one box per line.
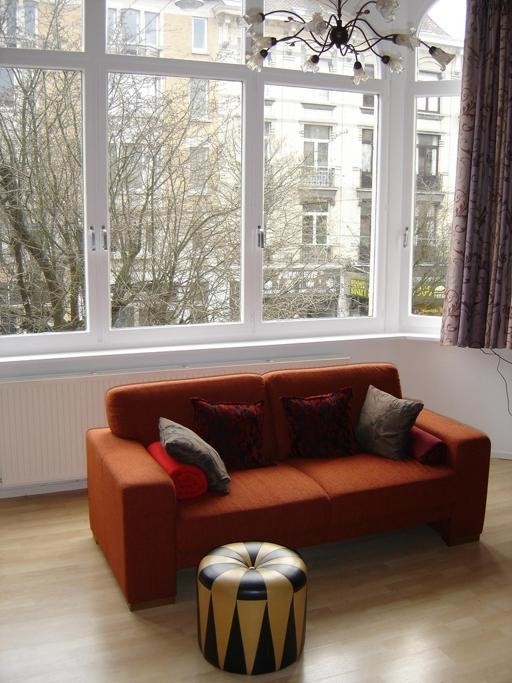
left=84, top=357, right=494, bottom=619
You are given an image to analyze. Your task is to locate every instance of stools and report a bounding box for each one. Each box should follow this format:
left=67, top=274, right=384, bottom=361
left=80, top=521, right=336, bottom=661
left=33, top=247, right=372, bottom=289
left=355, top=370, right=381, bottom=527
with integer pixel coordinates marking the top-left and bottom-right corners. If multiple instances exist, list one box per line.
left=194, top=536, right=314, bottom=678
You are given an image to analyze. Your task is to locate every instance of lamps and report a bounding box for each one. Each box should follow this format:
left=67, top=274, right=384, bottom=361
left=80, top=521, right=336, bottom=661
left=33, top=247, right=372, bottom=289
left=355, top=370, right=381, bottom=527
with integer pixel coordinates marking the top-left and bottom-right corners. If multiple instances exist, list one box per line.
left=238, top=0, right=459, bottom=93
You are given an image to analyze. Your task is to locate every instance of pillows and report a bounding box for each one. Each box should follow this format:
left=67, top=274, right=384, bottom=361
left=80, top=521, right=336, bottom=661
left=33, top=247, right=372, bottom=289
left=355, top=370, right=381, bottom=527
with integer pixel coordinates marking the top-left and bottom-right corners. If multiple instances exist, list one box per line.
left=158, top=413, right=237, bottom=497
left=351, top=380, right=427, bottom=464
left=194, top=394, right=276, bottom=472
left=280, top=385, right=360, bottom=460
left=145, top=439, right=207, bottom=500
